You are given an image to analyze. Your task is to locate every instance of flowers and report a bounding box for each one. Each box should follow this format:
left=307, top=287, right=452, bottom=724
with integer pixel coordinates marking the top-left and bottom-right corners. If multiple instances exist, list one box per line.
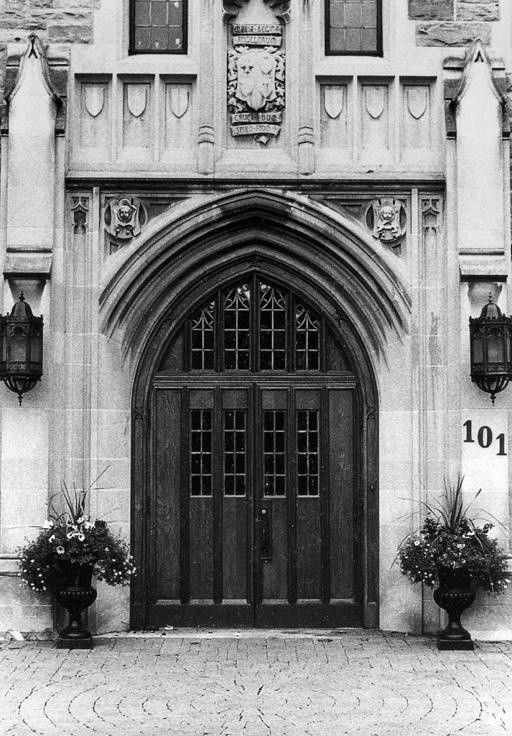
left=16, top=464, right=140, bottom=596
left=388, top=473, right=512, bottom=596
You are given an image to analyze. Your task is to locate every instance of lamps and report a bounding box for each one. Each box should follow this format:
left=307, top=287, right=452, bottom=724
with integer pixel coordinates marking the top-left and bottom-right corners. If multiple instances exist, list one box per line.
left=1, top=289, right=43, bottom=406
left=469, top=291, right=512, bottom=404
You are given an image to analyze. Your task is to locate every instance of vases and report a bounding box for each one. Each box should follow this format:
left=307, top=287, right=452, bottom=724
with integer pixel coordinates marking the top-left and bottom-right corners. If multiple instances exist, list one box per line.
left=433, top=556, right=476, bottom=649
left=55, top=562, right=96, bottom=649
